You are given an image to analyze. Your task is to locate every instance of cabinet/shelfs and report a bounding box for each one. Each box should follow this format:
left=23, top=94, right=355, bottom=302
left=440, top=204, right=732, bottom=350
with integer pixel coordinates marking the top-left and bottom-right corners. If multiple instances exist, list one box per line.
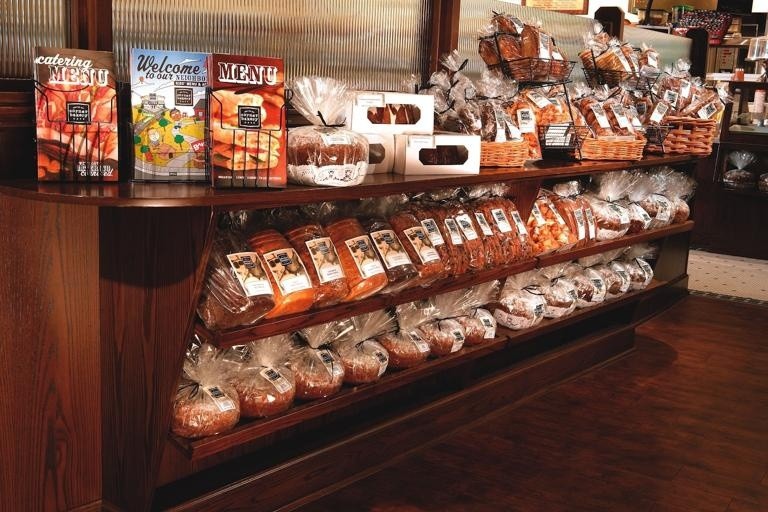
left=0, top=152, right=704, bottom=512
left=689, top=39, right=768, bottom=259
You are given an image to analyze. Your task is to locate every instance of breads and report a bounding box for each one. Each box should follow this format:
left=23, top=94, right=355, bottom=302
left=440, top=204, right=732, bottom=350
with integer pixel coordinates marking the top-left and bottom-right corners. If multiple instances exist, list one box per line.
left=196, top=174, right=695, bottom=331
left=403, top=12, right=728, bottom=142
left=286, top=127, right=368, bottom=188
left=172, top=245, right=659, bottom=438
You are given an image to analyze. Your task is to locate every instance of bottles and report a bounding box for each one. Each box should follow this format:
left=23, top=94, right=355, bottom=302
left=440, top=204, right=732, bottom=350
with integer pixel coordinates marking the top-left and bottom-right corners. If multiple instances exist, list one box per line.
left=678, top=9, right=728, bottom=38
left=752, top=90, right=766, bottom=122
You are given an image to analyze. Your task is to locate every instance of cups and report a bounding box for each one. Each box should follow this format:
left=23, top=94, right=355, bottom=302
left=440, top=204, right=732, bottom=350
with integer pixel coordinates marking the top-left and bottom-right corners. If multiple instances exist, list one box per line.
left=735, top=68, right=745, bottom=82
